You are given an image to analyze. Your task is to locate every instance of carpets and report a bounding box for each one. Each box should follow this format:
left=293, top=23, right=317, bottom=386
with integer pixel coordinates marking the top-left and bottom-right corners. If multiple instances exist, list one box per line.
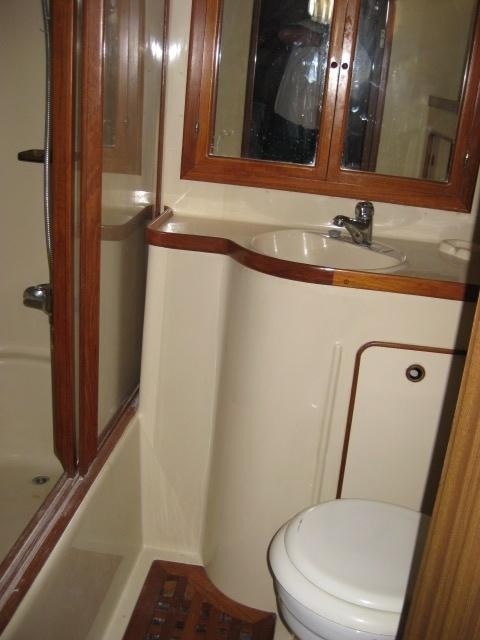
left=116, top=559, right=276, bottom=640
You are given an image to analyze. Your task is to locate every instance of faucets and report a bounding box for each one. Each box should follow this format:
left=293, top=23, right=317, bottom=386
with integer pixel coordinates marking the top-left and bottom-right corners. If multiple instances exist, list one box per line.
left=332, top=201, right=373, bottom=244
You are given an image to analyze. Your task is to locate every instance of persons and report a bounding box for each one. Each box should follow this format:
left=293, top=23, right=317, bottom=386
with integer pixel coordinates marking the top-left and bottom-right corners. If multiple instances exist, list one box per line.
left=260, top=0, right=372, bottom=168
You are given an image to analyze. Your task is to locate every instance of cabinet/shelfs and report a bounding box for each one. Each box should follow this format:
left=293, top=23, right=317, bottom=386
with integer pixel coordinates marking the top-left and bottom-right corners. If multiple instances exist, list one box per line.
left=180, top=0, right=479, bottom=212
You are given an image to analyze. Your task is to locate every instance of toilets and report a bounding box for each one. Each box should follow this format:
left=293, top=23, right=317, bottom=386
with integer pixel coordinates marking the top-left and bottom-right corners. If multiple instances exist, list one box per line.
left=267, top=498, right=432, bottom=640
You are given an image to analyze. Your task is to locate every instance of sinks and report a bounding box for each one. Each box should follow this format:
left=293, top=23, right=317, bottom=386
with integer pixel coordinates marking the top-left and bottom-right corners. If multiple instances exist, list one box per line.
left=251, top=228, right=407, bottom=273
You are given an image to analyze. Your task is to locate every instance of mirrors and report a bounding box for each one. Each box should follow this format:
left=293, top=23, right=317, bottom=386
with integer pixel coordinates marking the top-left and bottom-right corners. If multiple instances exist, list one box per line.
left=339, top=0, right=480, bottom=184
left=209, top=0, right=336, bottom=172
